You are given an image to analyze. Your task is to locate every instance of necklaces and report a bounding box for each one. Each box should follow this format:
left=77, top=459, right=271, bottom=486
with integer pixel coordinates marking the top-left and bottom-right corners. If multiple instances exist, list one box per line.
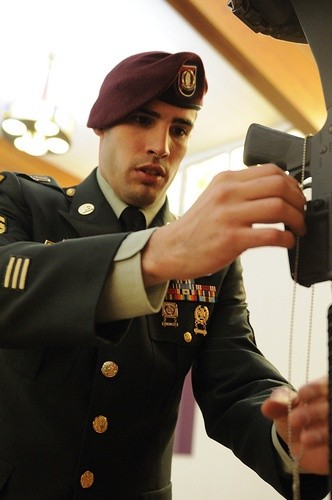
left=285, top=132, right=318, bottom=500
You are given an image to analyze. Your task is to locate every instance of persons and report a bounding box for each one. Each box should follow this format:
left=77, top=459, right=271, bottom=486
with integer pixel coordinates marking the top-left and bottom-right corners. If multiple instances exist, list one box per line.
left=0, top=50, right=332, bottom=500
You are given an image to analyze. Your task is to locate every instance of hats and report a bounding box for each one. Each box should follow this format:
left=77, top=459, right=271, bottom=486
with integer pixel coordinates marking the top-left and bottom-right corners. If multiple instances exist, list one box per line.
left=86, top=51, right=209, bottom=131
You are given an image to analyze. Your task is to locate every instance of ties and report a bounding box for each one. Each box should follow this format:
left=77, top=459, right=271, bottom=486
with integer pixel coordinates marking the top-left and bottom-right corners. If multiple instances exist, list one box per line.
left=118, top=206, right=146, bottom=234
